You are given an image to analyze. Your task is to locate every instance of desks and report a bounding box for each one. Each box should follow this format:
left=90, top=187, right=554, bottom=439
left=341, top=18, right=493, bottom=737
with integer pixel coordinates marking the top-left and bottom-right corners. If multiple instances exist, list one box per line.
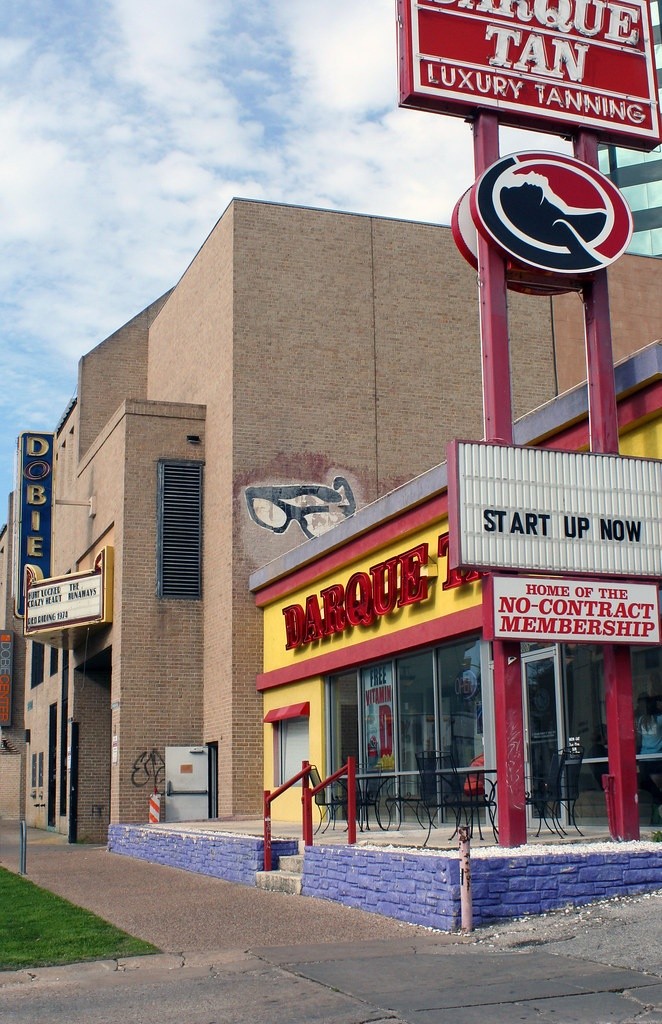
left=446, top=768, right=501, bottom=845
left=336, top=775, right=397, bottom=832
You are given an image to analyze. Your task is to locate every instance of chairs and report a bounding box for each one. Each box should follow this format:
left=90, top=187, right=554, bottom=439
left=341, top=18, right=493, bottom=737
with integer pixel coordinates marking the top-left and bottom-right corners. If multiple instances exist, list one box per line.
left=308, top=745, right=585, bottom=848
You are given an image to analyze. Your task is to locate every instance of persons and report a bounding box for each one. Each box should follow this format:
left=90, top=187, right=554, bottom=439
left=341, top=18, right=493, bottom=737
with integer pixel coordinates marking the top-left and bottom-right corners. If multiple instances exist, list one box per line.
left=585, top=722, right=607, bottom=792
left=636, top=691, right=662, bottom=816
